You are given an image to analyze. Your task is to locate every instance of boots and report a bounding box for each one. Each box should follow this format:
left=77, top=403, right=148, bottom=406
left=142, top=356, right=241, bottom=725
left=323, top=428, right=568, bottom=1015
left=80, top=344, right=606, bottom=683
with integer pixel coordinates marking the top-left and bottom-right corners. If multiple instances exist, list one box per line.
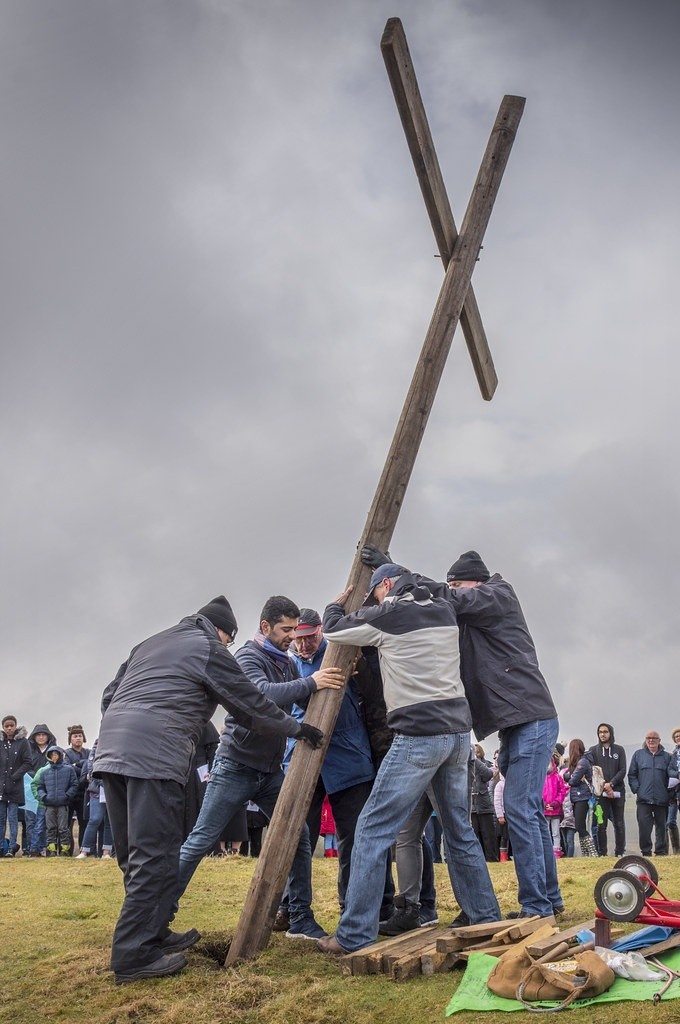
left=378, top=895, right=422, bottom=936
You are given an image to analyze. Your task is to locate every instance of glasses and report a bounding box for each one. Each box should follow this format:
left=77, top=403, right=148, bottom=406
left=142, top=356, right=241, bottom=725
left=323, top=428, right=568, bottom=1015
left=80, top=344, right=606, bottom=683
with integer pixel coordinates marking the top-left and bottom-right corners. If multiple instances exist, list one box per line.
left=294, top=629, right=320, bottom=643
left=226, top=632, right=235, bottom=649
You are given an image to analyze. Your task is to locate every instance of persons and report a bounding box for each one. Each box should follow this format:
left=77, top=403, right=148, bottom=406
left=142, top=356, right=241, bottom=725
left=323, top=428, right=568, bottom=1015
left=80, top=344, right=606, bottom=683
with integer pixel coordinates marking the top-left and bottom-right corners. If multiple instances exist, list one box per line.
left=0, top=706, right=680, bottom=864
left=166, top=595, right=346, bottom=942
left=92, top=595, right=325, bottom=985
left=319, top=541, right=564, bottom=954
left=272, top=608, right=396, bottom=932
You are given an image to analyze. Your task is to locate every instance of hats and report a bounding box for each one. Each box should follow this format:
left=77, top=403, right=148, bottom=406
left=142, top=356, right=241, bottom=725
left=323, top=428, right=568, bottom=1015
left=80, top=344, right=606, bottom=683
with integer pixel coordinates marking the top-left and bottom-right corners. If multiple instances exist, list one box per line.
left=362, top=564, right=409, bottom=607
left=446, top=550, right=491, bottom=582
left=196, top=594, right=238, bottom=640
left=294, top=608, right=321, bottom=638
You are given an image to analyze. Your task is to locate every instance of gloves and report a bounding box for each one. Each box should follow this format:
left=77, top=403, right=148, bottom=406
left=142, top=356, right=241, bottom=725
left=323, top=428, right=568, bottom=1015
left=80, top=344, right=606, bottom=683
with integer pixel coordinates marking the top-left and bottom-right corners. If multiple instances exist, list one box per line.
left=361, top=545, right=394, bottom=570
left=293, top=723, right=324, bottom=750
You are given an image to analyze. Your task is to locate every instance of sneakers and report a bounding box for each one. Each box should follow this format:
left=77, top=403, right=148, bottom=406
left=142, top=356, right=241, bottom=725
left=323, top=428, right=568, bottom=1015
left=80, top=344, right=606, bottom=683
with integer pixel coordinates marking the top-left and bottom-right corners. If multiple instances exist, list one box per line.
left=316, top=935, right=349, bottom=955
left=273, top=910, right=290, bottom=931
left=114, top=954, right=188, bottom=985
left=155, top=928, right=202, bottom=955
left=419, top=911, right=439, bottom=927
left=285, top=916, right=329, bottom=940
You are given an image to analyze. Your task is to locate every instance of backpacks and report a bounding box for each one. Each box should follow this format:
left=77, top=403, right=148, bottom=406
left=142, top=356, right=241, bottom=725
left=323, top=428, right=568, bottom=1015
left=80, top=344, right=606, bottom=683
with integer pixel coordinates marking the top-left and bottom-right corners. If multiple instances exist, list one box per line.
left=582, top=764, right=604, bottom=797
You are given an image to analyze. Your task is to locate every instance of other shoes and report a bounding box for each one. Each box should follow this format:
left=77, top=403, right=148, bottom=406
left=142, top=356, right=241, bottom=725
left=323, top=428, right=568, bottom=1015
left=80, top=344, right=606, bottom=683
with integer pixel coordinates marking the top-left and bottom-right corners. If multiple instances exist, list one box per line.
left=553, top=905, right=566, bottom=918
left=22, top=851, right=30, bottom=858
left=5, top=848, right=15, bottom=858
left=30, top=835, right=625, bottom=863
left=505, top=911, right=545, bottom=920
left=447, top=911, right=471, bottom=928
